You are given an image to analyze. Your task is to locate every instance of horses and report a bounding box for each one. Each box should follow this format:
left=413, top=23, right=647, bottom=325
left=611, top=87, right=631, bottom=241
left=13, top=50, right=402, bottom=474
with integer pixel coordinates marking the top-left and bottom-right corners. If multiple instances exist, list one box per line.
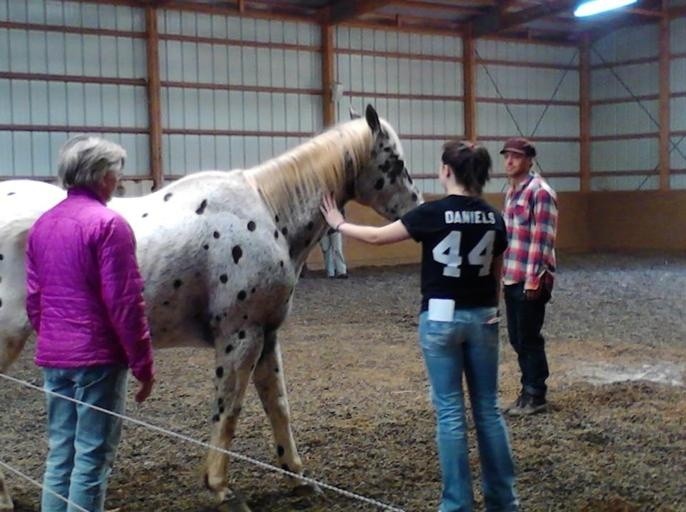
left=0, top=103, right=427, bottom=512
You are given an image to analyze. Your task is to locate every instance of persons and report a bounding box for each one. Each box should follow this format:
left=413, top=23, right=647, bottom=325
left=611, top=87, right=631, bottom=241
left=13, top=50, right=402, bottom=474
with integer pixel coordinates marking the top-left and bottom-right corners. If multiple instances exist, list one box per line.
left=317, top=227, right=352, bottom=279
left=492, top=139, right=560, bottom=417
left=23, top=136, right=154, bottom=511
left=315, top=138, right=520, bottom=511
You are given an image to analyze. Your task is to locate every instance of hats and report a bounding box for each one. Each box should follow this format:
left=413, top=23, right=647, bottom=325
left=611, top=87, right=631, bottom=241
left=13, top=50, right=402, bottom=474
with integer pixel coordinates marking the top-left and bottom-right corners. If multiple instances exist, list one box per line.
left=500, top=137, right=536, bottom=157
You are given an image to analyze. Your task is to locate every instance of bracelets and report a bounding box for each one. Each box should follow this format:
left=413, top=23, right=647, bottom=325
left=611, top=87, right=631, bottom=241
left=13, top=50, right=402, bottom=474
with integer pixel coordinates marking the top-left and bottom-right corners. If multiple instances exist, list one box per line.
left=336, top=219, right=348, bottom=229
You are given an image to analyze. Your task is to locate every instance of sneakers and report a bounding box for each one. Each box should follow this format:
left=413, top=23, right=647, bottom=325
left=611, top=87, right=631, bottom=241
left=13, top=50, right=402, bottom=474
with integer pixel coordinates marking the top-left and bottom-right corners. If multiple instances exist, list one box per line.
left=502, top=393, right=547, bottom=417
